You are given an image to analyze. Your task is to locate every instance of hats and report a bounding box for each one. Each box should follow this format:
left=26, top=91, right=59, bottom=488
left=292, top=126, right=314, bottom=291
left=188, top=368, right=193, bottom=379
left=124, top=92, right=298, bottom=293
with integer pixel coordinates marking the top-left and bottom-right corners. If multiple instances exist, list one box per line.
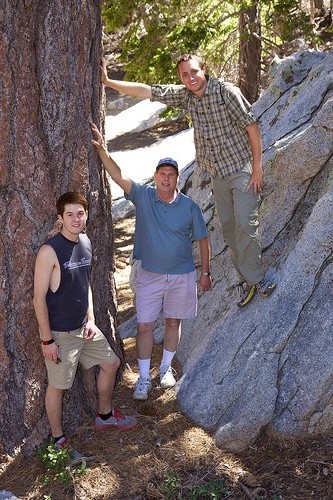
left=156, top=157, right=179, bottom=172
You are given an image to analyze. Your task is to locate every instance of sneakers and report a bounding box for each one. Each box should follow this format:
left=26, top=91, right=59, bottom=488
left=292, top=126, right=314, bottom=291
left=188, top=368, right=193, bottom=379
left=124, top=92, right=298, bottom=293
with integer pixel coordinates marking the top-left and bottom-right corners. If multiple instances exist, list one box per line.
left=234, top=280, right=256, bottom=306
left=92, top=406, right=137, bottom=431
left=132, top=376, right=153, bottom=400
left=255, top=276, right=275, bottom=297
left=159, top=363, right=178, bottom=388
left=50, top=435, right=82, bottom=465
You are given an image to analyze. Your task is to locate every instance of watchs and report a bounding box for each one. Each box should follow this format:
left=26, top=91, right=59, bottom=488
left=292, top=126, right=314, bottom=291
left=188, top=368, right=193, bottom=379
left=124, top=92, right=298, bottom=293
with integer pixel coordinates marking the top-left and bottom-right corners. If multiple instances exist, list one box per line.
left=201, top=271, right=210, bottom=277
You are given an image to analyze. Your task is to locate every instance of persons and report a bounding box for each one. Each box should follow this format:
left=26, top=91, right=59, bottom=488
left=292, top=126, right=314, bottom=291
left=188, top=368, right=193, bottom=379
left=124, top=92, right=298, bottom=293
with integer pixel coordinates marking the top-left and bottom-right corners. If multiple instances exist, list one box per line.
left=90, top=122, right=212, bottom=400
left=100, top=52, right=277, bottom=308
left=33, top=191, right=136, bottom=455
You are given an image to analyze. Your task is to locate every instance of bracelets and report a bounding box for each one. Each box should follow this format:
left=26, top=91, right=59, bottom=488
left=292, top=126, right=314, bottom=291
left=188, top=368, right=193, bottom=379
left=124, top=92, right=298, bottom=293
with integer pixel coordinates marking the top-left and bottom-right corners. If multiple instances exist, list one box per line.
left=42, top=338, right=54, bottom=345
